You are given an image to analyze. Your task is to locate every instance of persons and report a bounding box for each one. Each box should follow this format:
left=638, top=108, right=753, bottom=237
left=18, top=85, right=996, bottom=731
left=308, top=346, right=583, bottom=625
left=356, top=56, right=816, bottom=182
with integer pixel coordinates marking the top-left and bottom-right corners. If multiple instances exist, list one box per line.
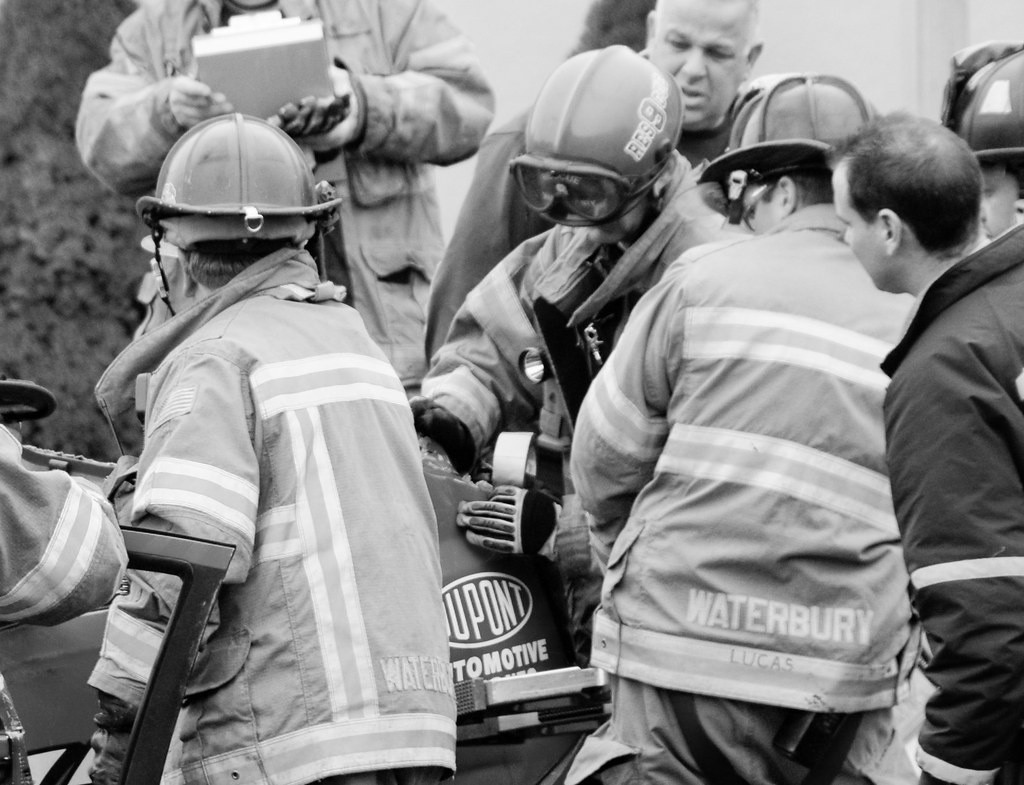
left=938, top=38, right=1024, bottom=138
left=73, top=107, right=463, bottom=785
left=414, top=434, right=627, bottom=784
left=423, top=0, right=670, bottom=363
left=413, top=40, right=743, bottom=709
left=543, top=71, right=938, bottom=785
left=827, top=111, right=1024, bottom=785
left=95, top=199, right=217, bottom=444
left=954, top=50, right=1024, bottom=244
left=0, top=676, right=42, bottom=783
left=2, top=367, right=159, bottom=783
left=629, top=1, right=773, bottom=196
left=73, top=4, right=494, bottom=408
left=621, top=73, right=832, bottom=354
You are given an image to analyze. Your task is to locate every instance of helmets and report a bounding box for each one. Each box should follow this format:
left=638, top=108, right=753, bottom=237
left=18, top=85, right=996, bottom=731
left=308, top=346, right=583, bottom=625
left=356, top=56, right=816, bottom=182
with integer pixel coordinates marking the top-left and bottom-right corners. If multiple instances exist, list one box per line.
left=136, top=110, right=341, bottom=316
left=510, top=45, right=680, bottom=229
left=696, top=74, right=878, bottom=225
left=939, top=40, right=1024, bottom=160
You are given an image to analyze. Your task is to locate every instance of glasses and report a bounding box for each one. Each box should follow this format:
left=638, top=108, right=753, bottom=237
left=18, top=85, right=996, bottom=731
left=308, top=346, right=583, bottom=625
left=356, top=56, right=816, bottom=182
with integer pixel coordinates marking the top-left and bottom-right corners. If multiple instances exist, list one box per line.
left=510, top=153, right=669, bottom=227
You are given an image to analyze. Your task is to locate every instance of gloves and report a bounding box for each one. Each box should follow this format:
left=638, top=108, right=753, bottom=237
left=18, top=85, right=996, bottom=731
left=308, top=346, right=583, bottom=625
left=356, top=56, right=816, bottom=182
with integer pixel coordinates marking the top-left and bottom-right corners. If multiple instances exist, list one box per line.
left=408, top=394, right=467, bottom=475
left=456, top=483, right=563, bottom=559
